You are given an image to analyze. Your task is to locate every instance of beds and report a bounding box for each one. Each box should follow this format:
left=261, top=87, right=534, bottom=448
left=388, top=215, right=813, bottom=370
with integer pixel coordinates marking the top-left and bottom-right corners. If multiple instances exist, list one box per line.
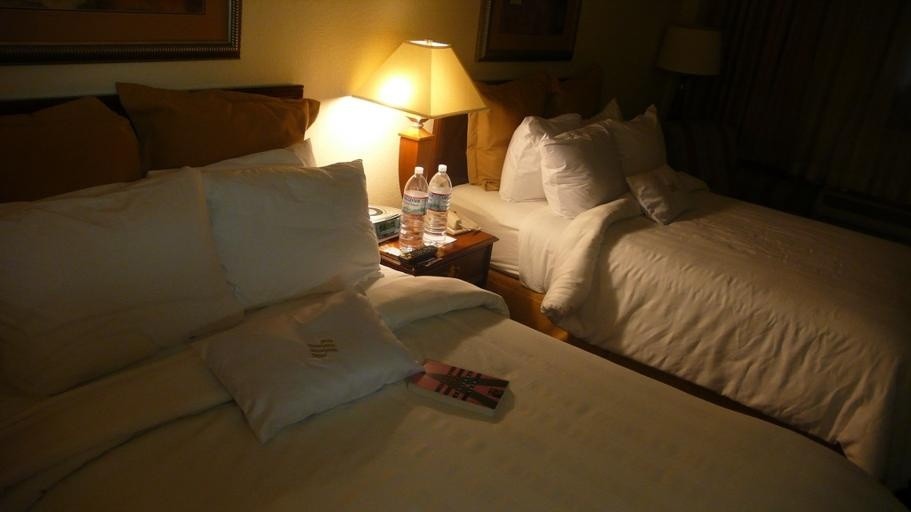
left=3, top=86, right=911, bottom=511
left=398, top=105, right=909, bottom=491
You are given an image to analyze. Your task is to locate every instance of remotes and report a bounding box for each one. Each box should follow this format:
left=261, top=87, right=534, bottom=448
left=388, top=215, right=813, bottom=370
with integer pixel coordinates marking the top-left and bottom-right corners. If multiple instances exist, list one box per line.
left=399, top=245, right=438, bottom=265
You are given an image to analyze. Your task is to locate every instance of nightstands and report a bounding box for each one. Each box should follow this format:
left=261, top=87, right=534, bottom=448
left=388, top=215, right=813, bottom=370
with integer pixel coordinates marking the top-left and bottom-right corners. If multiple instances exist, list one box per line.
left=379, top=208, right=498, bottom=290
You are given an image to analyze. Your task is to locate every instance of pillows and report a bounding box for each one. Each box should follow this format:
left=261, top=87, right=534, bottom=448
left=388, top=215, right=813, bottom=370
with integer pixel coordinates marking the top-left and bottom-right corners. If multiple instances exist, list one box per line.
left=465, top=66, right=694, bottom=227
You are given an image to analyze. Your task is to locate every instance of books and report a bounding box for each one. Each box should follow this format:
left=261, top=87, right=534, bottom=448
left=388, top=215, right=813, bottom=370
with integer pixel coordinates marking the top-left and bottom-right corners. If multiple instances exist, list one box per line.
left=406, top=358, right=511, bottom=417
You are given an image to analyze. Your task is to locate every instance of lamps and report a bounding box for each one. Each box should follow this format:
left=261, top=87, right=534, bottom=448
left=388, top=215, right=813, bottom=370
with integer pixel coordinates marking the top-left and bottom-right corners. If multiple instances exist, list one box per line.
left=352, top=40, right=485, bottom=206
left=656, top=22, right=726, bottom=122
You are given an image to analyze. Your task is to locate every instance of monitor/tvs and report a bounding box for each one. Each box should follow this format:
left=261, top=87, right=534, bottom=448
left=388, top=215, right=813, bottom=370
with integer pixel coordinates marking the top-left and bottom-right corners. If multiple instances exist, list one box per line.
left=472, top=0, right=583, bottom=81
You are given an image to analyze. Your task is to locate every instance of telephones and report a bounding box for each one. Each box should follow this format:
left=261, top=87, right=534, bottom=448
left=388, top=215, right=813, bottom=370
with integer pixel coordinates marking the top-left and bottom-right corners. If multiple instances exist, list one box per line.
left=446, top=209, right=481, bottom=236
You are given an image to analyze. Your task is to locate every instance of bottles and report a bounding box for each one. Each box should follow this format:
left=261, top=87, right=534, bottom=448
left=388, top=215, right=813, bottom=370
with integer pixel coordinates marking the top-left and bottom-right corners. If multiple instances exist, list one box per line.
left=399, top=166, right=429, bottom=252
left=425, top=164, right=452, bottom=246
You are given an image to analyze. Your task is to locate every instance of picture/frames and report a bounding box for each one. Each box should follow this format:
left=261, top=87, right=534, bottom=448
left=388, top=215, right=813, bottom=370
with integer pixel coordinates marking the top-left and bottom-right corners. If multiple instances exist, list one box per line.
left=1, top=0, right=243, bottom=65
left=475, top=1, right=584, bottom=62
